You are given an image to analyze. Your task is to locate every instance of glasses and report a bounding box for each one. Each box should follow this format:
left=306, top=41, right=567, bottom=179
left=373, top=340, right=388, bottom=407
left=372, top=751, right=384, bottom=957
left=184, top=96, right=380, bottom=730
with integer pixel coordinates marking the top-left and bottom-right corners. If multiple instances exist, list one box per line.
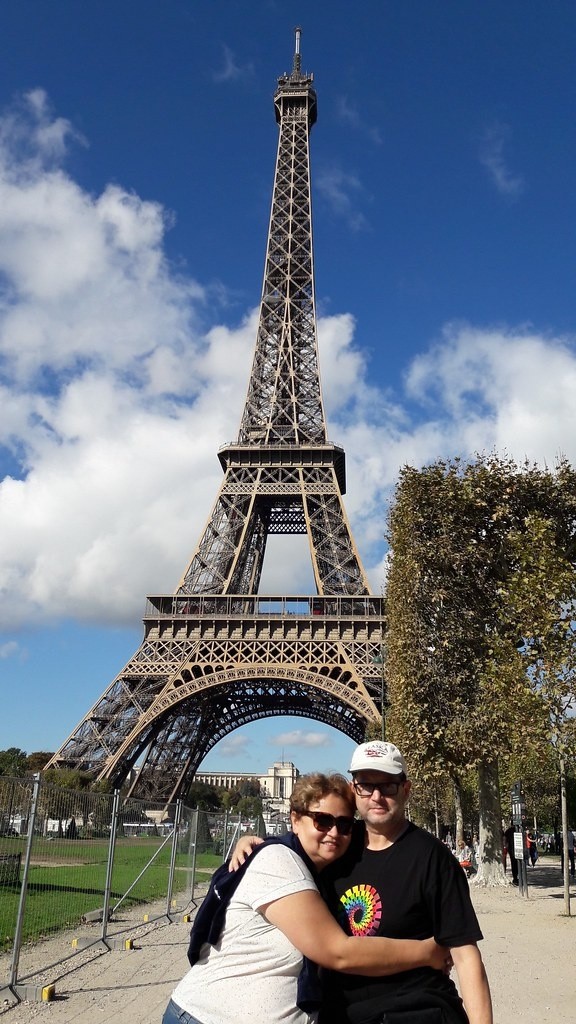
left=293, top=807, right=358, bottom=836
left=351, top=773, right=403, bottom=796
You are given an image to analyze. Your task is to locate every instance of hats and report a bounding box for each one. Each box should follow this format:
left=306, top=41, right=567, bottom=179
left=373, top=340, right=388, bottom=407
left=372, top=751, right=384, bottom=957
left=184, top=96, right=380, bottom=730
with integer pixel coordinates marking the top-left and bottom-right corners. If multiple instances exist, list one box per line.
left=350, top=741, right=408, bottom=782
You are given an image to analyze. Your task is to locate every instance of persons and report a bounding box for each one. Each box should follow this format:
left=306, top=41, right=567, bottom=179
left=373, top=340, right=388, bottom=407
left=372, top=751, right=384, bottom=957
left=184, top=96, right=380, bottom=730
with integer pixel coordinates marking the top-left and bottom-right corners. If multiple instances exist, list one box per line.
left=157, top=772, right=454, bottom=1024
left=229, top=740, right=493, bottom=1024
left=444, top=833, right=479, bottom=878
left=503, top=817, right=576, bottom=885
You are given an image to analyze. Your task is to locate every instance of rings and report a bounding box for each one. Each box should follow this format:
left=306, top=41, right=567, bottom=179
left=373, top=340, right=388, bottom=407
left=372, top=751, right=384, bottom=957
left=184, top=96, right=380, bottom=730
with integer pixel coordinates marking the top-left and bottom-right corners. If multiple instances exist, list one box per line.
left=444, top=958, right=451, bottom=966
